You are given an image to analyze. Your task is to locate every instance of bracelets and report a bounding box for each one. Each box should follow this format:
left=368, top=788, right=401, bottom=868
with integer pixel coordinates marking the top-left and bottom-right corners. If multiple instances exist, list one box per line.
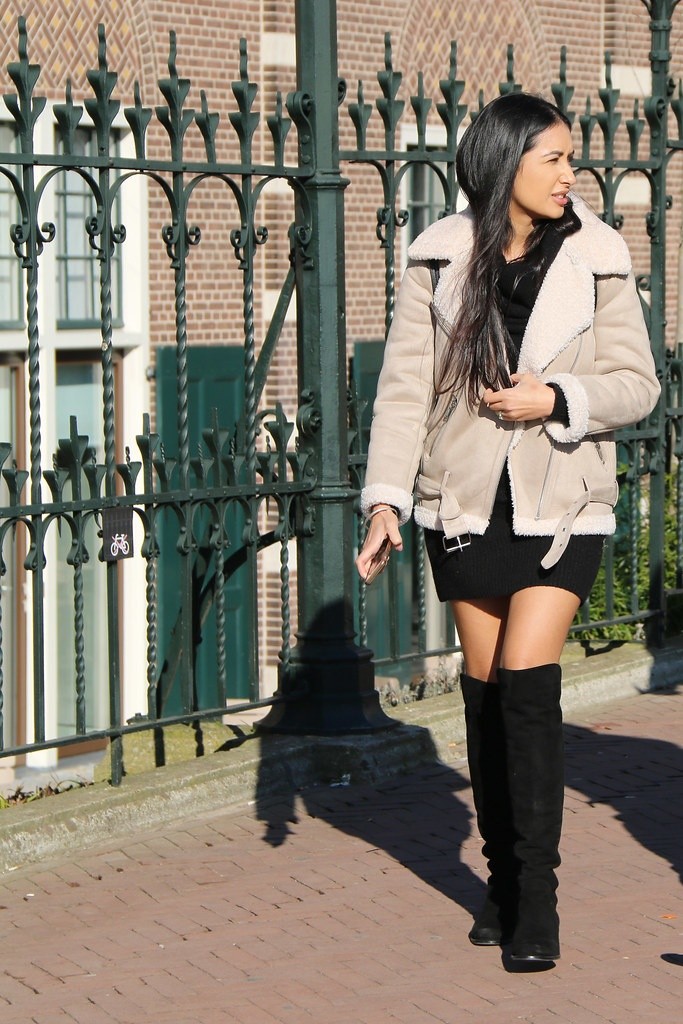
left=363, top=506, right=398, bottom=531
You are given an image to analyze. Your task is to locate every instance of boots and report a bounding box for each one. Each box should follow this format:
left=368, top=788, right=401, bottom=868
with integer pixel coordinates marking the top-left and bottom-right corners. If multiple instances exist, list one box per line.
left=460, top=673, right=519, bottom=945
left=496, top=663, right=564, bottom=961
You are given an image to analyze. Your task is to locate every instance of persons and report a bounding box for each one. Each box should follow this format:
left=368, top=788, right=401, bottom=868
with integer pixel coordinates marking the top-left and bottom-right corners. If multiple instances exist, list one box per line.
left=360, top=92, right=662, bottom=967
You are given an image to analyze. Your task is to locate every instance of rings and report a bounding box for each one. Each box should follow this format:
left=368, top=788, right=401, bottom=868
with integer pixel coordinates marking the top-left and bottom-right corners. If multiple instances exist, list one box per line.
left=498, top=411, right=504, bottom=421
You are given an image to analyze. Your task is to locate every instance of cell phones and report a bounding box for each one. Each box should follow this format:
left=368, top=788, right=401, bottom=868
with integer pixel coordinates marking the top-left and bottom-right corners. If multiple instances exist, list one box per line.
left=365, top=537, right=392, bottom=585
